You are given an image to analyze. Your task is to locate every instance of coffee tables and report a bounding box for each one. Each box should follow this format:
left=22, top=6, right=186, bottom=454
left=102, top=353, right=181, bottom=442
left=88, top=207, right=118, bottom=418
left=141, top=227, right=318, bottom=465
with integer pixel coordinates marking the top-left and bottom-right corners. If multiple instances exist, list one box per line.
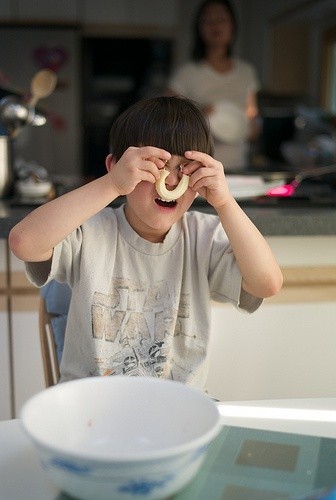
left=0, top=417, right=335, bottom=500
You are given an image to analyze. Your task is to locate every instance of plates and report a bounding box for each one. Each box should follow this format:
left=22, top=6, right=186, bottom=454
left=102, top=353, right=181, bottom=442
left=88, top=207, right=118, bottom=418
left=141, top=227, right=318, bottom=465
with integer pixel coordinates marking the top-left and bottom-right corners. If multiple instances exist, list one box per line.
left=207, top=100, right=246, bottom=143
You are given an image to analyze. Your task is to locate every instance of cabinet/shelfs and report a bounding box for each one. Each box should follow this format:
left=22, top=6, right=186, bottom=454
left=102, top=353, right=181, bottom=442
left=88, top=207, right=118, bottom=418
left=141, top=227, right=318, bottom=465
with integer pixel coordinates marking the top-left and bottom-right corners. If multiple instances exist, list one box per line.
left=81, top=20, right=179, bottom=178
left=1, top=22, right=80, bottom=194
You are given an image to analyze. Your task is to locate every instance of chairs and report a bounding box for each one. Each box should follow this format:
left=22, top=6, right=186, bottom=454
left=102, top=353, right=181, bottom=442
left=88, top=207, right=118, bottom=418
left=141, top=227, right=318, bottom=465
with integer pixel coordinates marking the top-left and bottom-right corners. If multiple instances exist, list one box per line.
left=39, top=294, right=60, bottom=391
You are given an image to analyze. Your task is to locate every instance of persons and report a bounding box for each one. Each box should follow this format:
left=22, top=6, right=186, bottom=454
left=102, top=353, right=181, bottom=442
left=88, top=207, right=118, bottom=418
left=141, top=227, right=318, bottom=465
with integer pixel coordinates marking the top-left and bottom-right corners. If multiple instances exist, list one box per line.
left=163, top=0, right=262, bottom=177
left=9, top=93, right=284, bottom=405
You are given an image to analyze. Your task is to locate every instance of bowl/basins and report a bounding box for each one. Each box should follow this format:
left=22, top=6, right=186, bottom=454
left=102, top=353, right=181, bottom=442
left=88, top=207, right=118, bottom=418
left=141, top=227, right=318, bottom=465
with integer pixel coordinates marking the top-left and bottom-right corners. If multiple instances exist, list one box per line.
left=19, top=375, right=223, bottom=500
